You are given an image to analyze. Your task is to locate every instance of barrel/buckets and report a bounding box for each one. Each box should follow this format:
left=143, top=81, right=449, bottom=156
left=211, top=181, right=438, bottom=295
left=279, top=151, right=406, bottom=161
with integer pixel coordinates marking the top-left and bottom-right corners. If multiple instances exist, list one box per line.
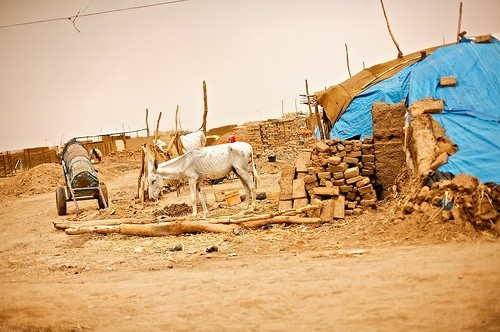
left=225, top=190, right=241, bottom=207
left=268, top=153, right=276, bottom=162
left=214, top=190, right=225, bottom=202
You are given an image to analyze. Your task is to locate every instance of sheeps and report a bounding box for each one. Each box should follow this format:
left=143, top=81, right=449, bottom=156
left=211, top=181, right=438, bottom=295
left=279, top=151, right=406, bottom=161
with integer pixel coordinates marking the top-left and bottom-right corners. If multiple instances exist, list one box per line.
left=147, top=142, right=261, bottom=219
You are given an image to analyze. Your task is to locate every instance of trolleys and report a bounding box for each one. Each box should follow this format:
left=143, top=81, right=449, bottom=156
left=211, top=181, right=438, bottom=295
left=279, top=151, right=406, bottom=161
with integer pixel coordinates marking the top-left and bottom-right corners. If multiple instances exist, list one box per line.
left=56, top=158, right=109, bottom=215
